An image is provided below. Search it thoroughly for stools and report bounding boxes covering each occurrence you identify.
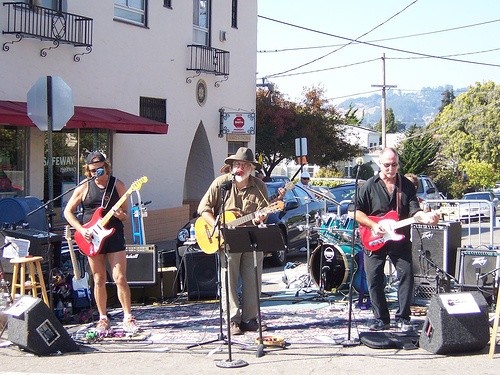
[9,256,50,308]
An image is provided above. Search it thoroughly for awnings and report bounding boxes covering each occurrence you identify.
[0,100,168,134]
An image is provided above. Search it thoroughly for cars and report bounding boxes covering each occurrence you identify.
[457,192,499,221]
[175,180,326,266]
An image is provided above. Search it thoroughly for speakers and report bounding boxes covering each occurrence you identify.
[456,246,500,300]
[103,243,157,284]
[419,290,490,354]
[183,251,220,299]
[7,295,81,354]
[410,222,461,306]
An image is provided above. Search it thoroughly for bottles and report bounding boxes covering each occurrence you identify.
[57,298,64,318]
[189,224,195,238]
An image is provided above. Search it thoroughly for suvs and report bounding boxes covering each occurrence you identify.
[414,175,441,212]
[272,175,290,183]
[324,185,359,215]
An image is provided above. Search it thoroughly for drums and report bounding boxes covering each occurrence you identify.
[309,241,362,291]
[318,211,342,244]
[338,212,361,245]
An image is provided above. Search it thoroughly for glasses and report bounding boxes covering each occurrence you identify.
[90,164,105,173]
[302,178,310,180]
[383,162,398,168]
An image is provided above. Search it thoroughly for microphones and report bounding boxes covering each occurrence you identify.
[357,159,362,171]
[221,181,232,190]
[96,168,104,176]
[475,268,480,284]
[231,169,244,176]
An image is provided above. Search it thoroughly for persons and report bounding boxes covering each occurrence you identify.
[347,147,439,331]
[197,147,286,335]
[64,152,143,333]
[404,173,418,186]
[0,175,21,191]
[296,170,322,198]
[256,171,263,180]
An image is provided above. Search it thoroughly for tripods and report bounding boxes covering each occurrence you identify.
[286,200,313,288]
[186,178,248,349]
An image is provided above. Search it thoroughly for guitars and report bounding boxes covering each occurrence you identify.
[64,223,93,309]
[194,200,287,256]
[353,249,387,297]
[357,206,457,253]
[74,175,149,258]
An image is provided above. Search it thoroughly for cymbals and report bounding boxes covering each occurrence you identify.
[306,188,343,207]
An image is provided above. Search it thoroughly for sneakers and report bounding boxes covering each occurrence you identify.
[94,317,112,332]
[122,314,141,333]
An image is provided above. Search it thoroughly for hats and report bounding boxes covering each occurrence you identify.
[224,146,259,166]
[86,151,105,165]
[300,171,310,178]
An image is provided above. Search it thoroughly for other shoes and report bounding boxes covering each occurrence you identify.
[368,319,390,331]
[230,321,243,335]
[396,318,412,327]
[240,319,268,332]
